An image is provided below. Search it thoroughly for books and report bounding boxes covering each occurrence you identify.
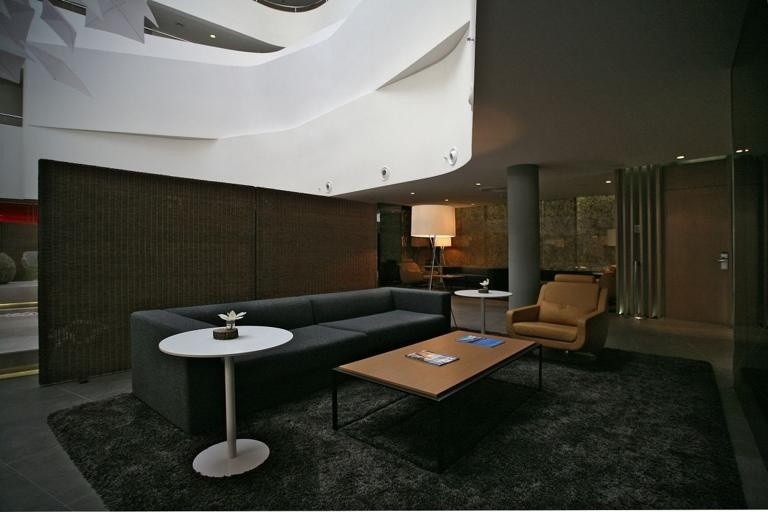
[456,332,507,348]
[404,349,459,367]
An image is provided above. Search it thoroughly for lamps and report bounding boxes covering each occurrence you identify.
[411,202,463,294]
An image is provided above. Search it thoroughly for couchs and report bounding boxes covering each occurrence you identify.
[131,286,455,426]
[506,274,617,364]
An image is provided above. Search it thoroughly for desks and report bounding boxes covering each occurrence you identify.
[330,330,544,425]
[452,287,514,336]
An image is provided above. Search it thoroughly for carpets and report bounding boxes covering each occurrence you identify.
[40,346,742,509]
